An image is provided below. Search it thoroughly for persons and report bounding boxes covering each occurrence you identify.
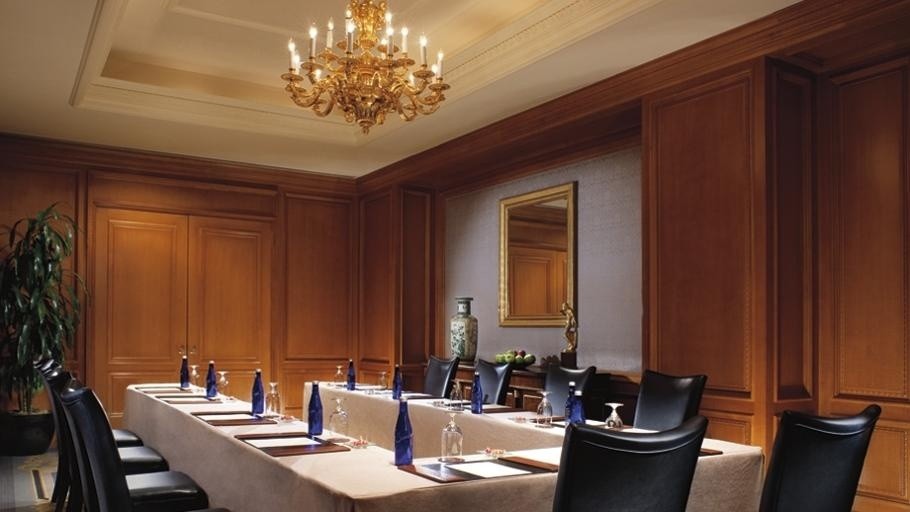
[560,303,580,350]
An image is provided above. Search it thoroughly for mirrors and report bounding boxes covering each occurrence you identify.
[497,181,579,328]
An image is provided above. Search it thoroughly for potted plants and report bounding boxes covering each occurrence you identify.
[0,197,91,458]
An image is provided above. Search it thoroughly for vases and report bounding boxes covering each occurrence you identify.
[449,296,479,365]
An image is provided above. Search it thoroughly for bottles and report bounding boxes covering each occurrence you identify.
[393,364,402,400]
[570,390,585,424]
[395,395,413,465]
[180,356,189,387]
[471,372,482,414]
[347,359,355,390]
[252,369,264,413]
[308,380,323,435]
[565,382,576,425]
[206,361,217,397]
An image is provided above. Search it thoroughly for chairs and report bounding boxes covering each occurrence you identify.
[477,358,516,404]
[757,402,880,509]
[552,413,711,512]
[541,361,599,413]
[423,356,461,395]
[632,368,708,430]
[29,351,210,512]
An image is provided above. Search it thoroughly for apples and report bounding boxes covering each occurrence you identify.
[495,349,536,365]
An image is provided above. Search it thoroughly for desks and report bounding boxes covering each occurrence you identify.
[424,392,763,457]
[422,448,765,512]
[124,380,766,511]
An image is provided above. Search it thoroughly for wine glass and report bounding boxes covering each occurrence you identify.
[604,402,624,432]
[334,365,344,389]
[536,391,553,427]
[377,371,388,395]
[189,365,201,392]
[440,413,463,462]
[264,382,282,415]
[217,371,231,402]
[449,380,464,411]
[327,397,350,440]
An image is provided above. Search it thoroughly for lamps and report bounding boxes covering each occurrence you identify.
[280,1,450,138]
